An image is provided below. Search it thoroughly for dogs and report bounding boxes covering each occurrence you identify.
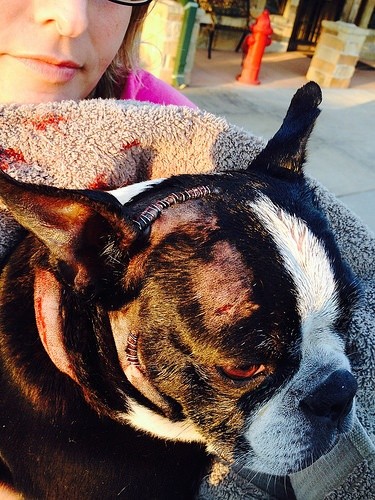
[0,81,362,500]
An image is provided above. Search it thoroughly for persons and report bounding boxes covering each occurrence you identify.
[1,0,206,121]
